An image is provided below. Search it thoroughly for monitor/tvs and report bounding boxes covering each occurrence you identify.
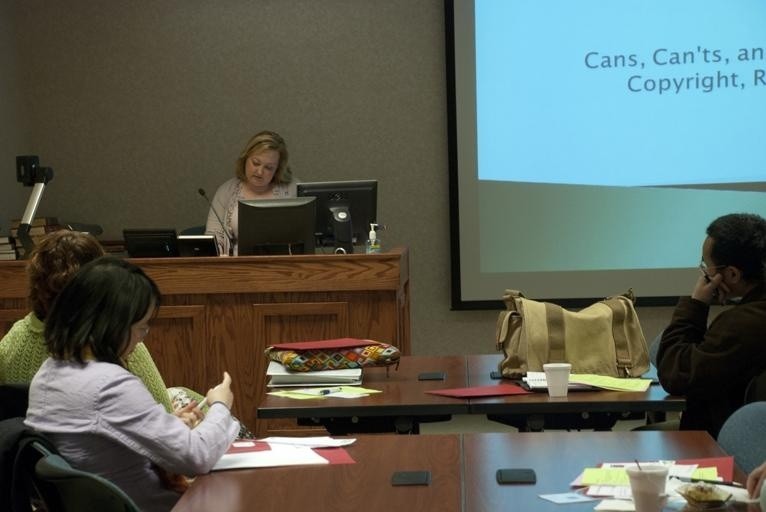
[122,228,182,259]
[238,196,317,256]
[297,180,377,254]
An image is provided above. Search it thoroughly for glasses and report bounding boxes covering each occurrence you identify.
[699,256,725,279]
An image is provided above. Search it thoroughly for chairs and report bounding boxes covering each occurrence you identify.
[649,321,711,368]
[0,383,29,420]
[717,400,766,475]
[24,441,138,512]
[179,224,206,235]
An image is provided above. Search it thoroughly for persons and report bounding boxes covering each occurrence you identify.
[631,213,766,441]
[748,461,765,512]
[21,254,243,512]
[0,227,254,475]
[206,131,305,257]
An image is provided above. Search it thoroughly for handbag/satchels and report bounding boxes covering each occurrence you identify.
[496,289,649,379]
[264,339,400,372]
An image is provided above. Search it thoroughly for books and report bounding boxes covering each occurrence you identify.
[1,217,59,259]
[266,360,363,387]
[517,370,592,391]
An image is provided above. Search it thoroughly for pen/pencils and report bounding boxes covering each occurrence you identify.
[669,476,743,487]
[701,270,719,298]
[198,398,207,410]
[320,388,342,395]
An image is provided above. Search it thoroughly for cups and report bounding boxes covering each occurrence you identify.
[541,360,572,398]
[624,463,671,511]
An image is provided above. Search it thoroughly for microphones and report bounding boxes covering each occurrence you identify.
[198,188,233,256]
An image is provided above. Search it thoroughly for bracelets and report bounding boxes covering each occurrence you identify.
[212,400,231,410]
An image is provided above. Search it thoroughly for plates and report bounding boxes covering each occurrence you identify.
[652,492,738,510]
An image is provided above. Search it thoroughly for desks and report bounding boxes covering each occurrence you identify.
[468,353,685,433]
[256,353,468,434]
[463,430,749,512]
[171,434,462,511]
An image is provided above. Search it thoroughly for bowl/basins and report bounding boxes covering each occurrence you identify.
[675,482,732,506]
